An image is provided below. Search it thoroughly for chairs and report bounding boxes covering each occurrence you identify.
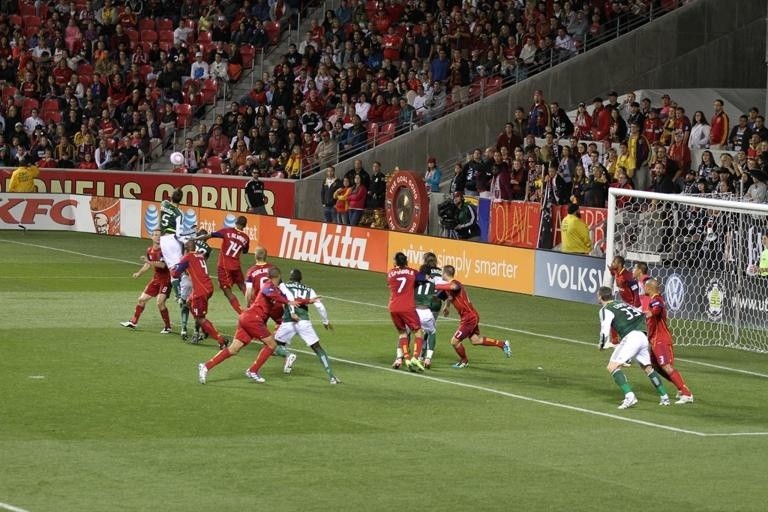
[1,2,287,127]
[471,76,502,98]
[361,0,407,41]
[367,122,397,146]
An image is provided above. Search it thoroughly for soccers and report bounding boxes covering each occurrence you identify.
[169,151,185,166]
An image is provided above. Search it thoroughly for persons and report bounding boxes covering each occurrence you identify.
[639,280,694,405]
[274,269,341,383]
[594,283,670,409]
[199,266,321,382]
[1,1,767,371]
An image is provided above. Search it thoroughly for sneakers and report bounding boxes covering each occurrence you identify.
[392,340,512,373]
[618,385,694,410]
[121,321,342,385]
